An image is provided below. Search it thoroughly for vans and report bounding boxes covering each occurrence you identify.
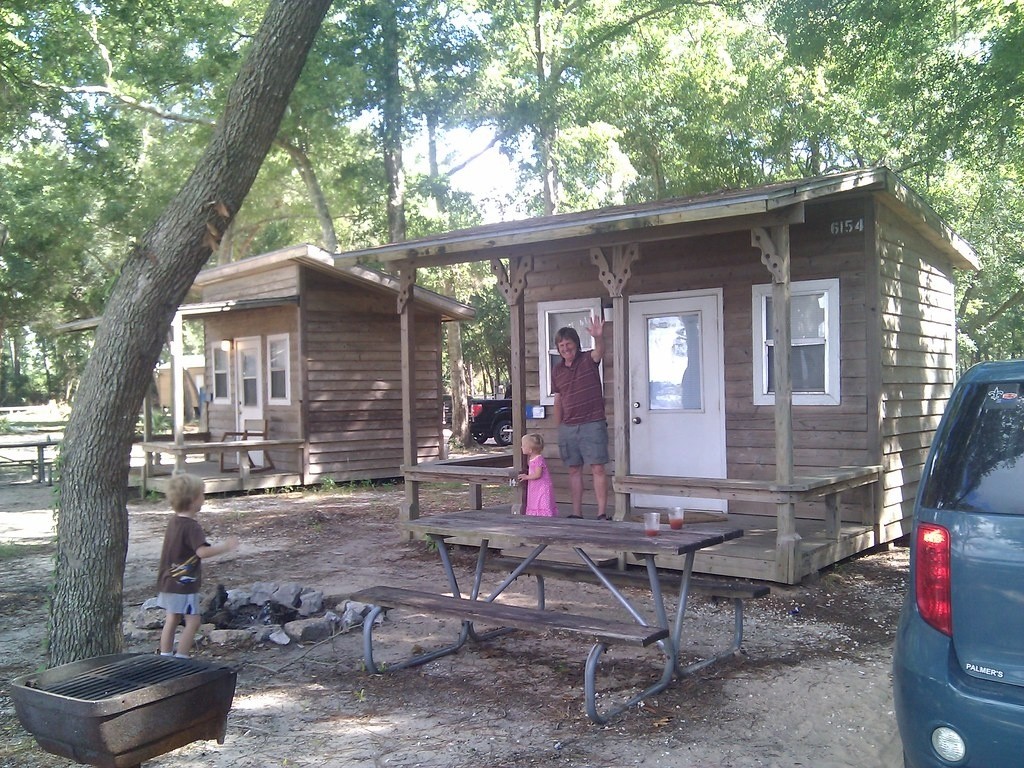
[892,358,1024,768]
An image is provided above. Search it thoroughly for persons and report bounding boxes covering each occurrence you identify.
[158,471,243,660]
[517,433,559,518]
[550,314,610,521]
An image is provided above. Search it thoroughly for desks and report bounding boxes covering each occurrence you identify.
[0,440,62,484]
[392,507,744,686]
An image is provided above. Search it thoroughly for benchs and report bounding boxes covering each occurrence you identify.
[350,585,669,724]
[477,557,771,677]
[0,459,55,487]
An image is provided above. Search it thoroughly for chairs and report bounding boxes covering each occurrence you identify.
[220,418,276,473]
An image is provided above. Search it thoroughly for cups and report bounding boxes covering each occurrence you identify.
[668,507,684,530]
[643,512,661,537]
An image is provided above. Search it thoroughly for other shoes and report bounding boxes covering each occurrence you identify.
[597,514,608,520]
[567,514,583,519]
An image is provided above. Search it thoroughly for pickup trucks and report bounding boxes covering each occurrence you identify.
[442,382,512,448]
